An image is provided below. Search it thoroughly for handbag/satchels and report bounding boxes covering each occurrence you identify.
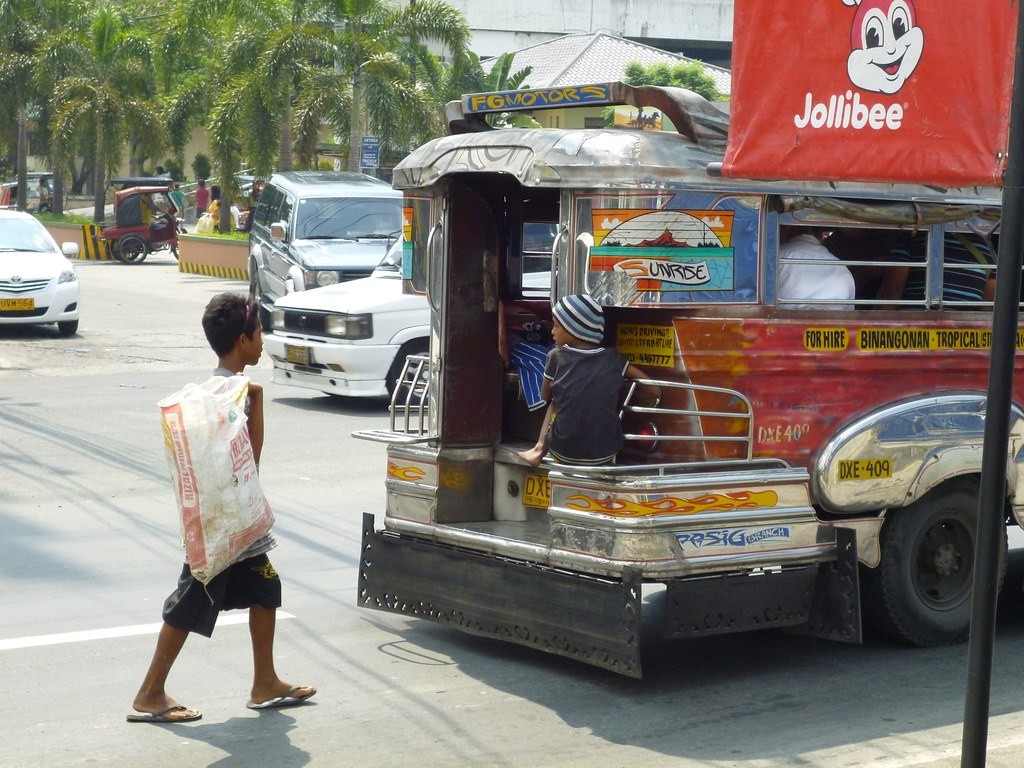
[195,213,214,233]
[159,375,274,585]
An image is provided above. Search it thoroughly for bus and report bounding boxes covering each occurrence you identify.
[358,81,1024,679]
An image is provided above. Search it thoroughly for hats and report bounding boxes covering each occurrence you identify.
[551,294,605,345]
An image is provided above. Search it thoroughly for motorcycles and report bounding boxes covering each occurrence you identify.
[100,185,188,264]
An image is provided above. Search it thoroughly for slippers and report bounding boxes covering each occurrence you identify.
[508,449,534,469]
[127,706,203,721]
[247,686,317,708]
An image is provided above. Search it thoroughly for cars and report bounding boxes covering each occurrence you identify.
[0,208,82,336]
[264,230,431,410]
[19,171,264,232]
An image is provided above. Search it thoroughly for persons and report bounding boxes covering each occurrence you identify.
[127,291,318,723]
[498,269,555,410]
[142,196,163,231]
[167,184,185,220]
[38,179,49,201]
[155,166,166,179]
[193,179,209,219]
[214,200,241,232]
[778,225,856,310]
[874,230,998,312]
[208,185,221,230]
[825,225,895,309]
[518,292,665,469]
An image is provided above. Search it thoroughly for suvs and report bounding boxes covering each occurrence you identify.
[246,171,407,333]
